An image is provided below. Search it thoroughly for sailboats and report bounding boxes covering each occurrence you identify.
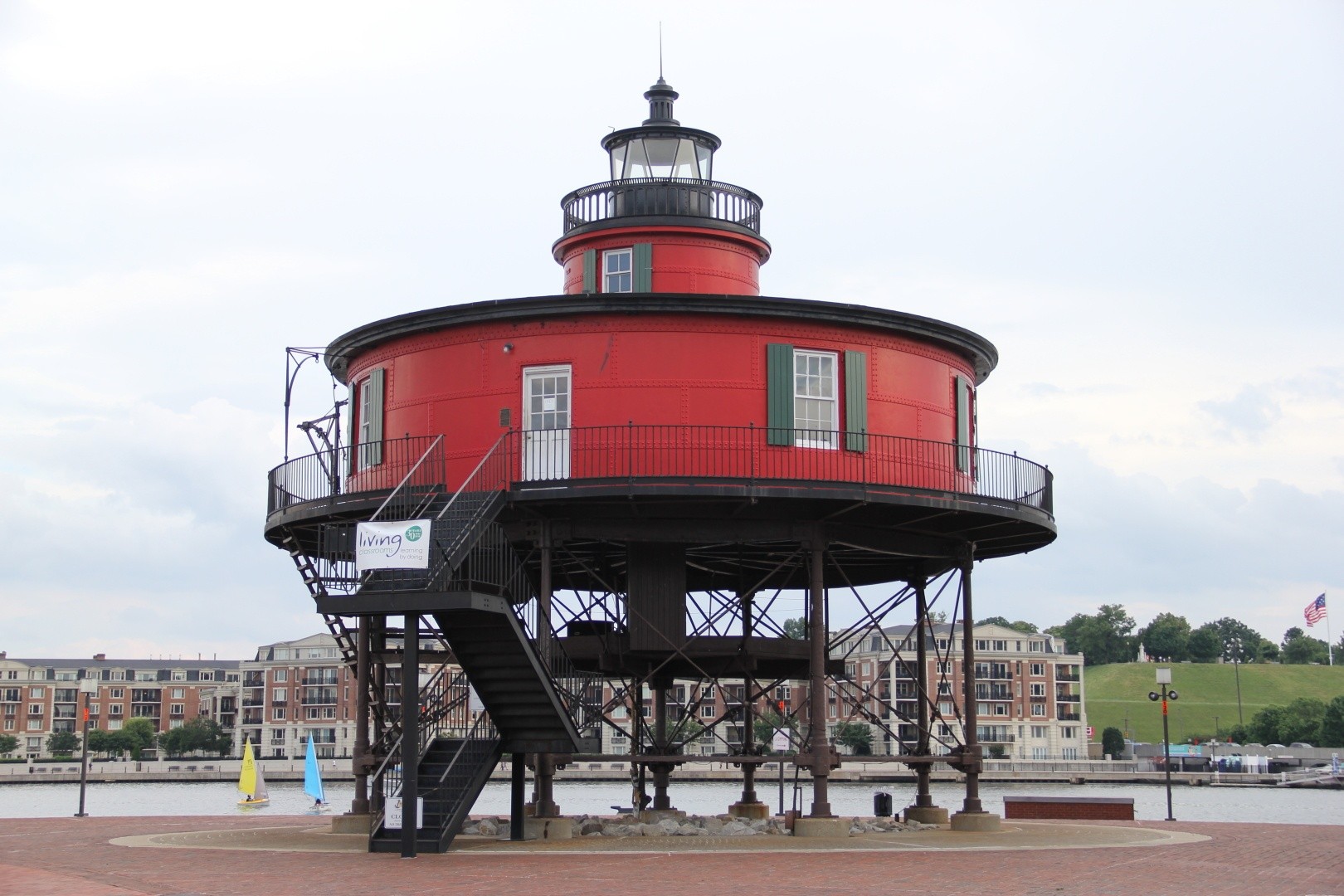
[236,735,271,808]
[303,730,332,813]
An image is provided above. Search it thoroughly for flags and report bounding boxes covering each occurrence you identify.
[1304,593,1327,627]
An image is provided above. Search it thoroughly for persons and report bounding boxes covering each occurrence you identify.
[247,795,253,802]
[89,762,92,772]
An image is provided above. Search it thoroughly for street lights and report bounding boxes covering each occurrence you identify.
[1148,668,1179,822]
[74,677,99,817]
